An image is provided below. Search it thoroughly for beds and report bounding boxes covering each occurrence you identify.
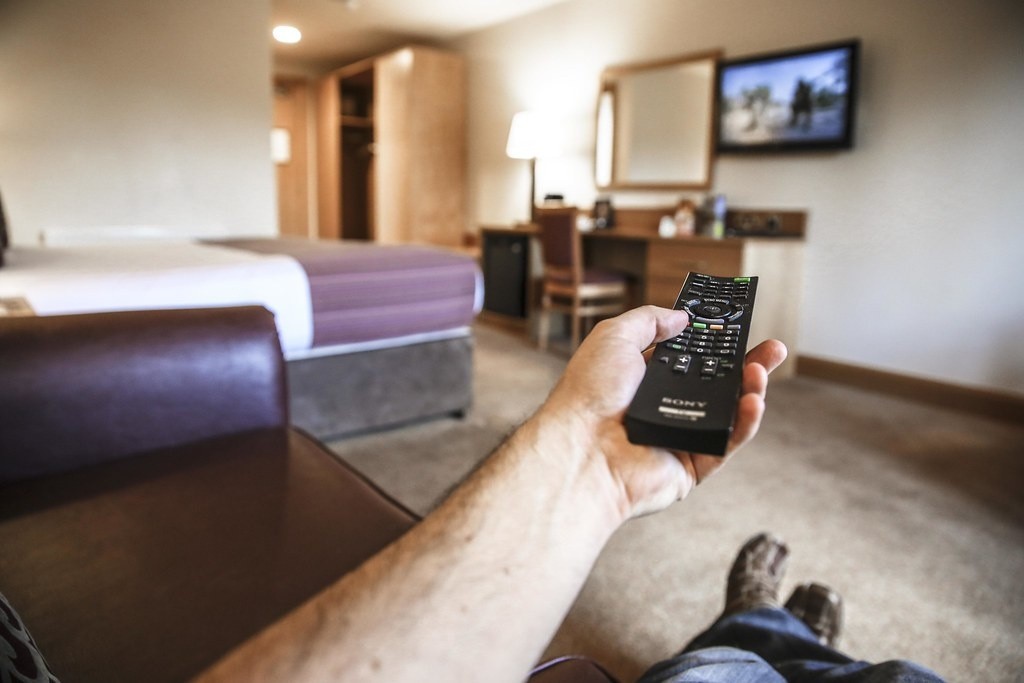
[0,191,486,439]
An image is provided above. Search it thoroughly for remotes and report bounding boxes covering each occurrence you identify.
[621,269,758,458]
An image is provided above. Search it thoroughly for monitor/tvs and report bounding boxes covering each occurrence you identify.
[706,35,863,154]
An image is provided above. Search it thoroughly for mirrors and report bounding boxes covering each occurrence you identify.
[593,48,725,195]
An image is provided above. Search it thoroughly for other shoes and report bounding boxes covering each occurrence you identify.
[784,584,840,650]
[725,534,788,612]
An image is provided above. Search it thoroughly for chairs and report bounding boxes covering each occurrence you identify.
[527,200,628,359]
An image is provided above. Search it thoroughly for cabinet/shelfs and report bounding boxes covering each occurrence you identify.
[315,37,470,251]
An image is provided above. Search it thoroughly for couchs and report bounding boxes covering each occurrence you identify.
[0,302,617,683]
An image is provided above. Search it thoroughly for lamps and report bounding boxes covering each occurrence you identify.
[498,107,555,230]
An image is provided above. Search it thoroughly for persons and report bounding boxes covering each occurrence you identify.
[191,305,945,683]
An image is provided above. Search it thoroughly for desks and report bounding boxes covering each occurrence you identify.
[513,201,742,384]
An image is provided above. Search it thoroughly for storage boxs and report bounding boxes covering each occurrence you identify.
[474,226,527,324]
